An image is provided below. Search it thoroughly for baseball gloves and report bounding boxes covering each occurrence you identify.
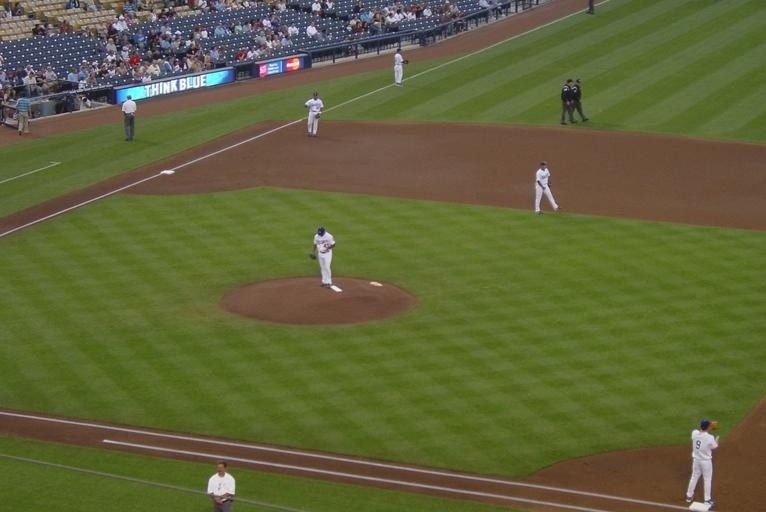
[315,113,320,118]
[308,253,317,260]
[404,60,408,64]
[711,421,719,429]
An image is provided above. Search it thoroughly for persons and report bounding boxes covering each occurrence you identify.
[0,0,206,137]
[304,92,324,138]
[534,160,561,215]
[560,79,576,125]
[310,227,335,288]
[393,47,408,87]
[204,1,498,65]
[586,0,597,16]
[121,96,136,142]
[570,79,590,123]
[686,420,721,503]
[205,460,237,512]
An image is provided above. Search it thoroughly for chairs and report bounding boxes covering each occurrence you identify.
[0,1,488,86]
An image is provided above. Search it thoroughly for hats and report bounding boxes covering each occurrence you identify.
[700,419,712,431]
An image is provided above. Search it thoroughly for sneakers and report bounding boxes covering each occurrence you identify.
[704,500,715,510]
[686,496,693,503]
